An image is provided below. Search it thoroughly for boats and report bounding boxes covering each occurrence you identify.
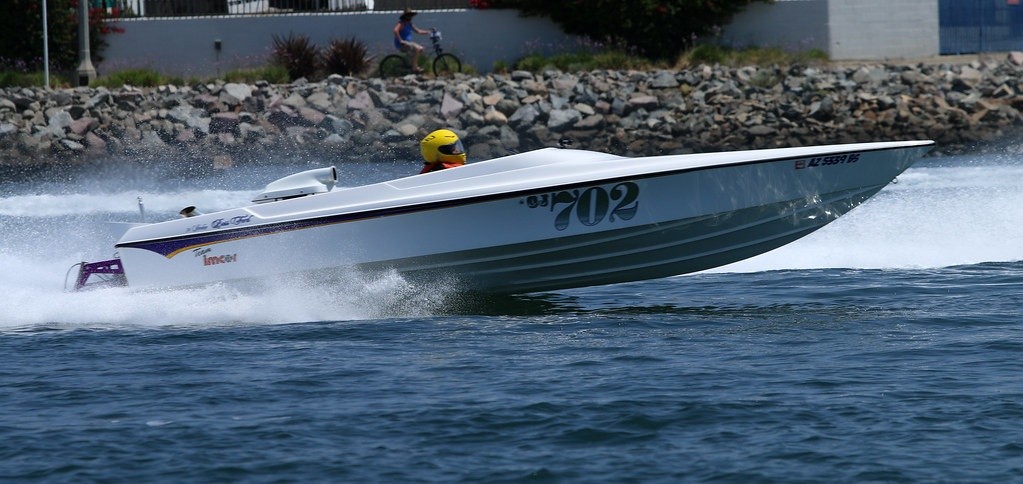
[76,141,936,298]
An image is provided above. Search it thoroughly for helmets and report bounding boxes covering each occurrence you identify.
[419,129,466,164]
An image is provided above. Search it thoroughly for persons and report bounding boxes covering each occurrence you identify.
[393,7,432,74]
[419,129,467,175]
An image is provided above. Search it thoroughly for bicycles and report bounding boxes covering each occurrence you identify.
[375,29,466,81]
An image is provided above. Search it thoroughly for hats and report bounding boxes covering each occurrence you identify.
[400,7,417,20]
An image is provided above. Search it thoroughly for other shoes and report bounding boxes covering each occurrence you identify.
[412,67,424,74]
